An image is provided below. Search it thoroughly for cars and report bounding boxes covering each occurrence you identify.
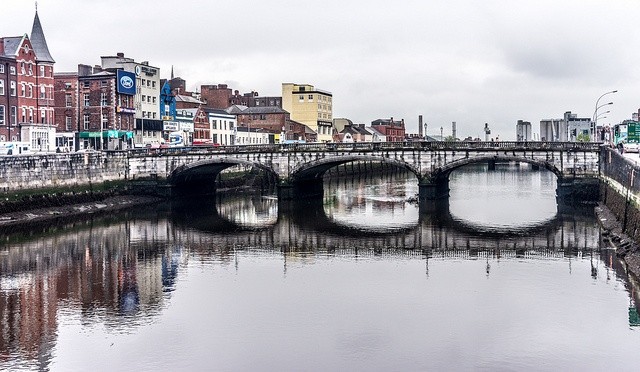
[624,144,639,153]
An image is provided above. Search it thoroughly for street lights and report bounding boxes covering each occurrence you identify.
[595,116,607,142]
[595,111,610,142]
[595,90,617,142]
[593,102,613,120]
